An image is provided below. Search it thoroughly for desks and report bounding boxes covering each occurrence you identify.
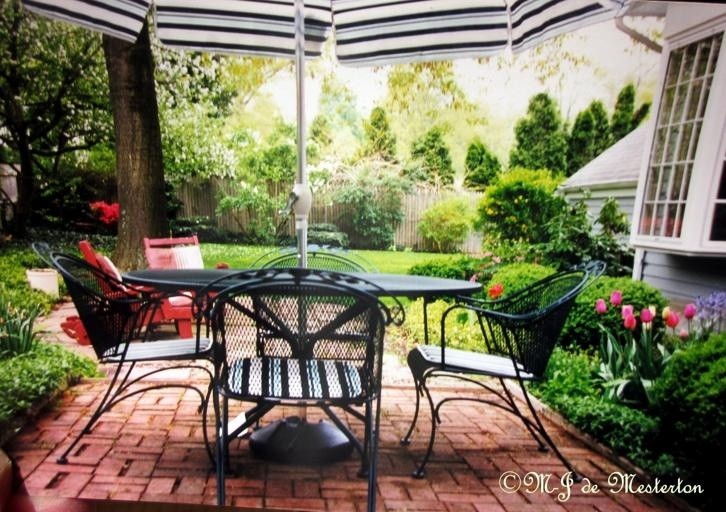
[120,267,481,464]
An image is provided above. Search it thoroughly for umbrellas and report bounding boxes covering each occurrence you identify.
[22,0,634,422]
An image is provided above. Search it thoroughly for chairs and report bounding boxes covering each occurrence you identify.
[79,234,226,338]
[192,268,392,512]
[400,260,606,481]
[49,249,224,470]
[250,244,404,358]
